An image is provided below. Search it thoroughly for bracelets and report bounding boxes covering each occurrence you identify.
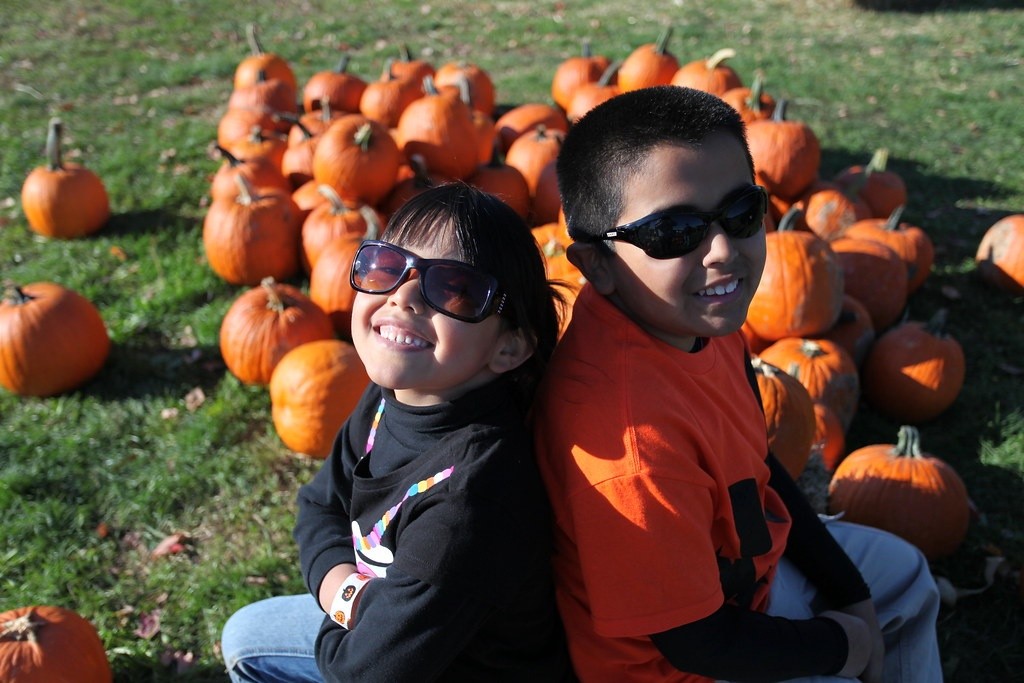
[329,572,375,632]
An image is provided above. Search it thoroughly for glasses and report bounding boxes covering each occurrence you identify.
[350,240,523,326]
[586,185,768,260]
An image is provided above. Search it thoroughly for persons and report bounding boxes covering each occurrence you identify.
[224,182,575,683]
[538,87,941,683]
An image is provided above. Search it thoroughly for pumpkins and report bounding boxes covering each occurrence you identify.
[21,118,112,239]
[0,281,110,396]
[0,604,114,683]
[204,22,1024,571]
